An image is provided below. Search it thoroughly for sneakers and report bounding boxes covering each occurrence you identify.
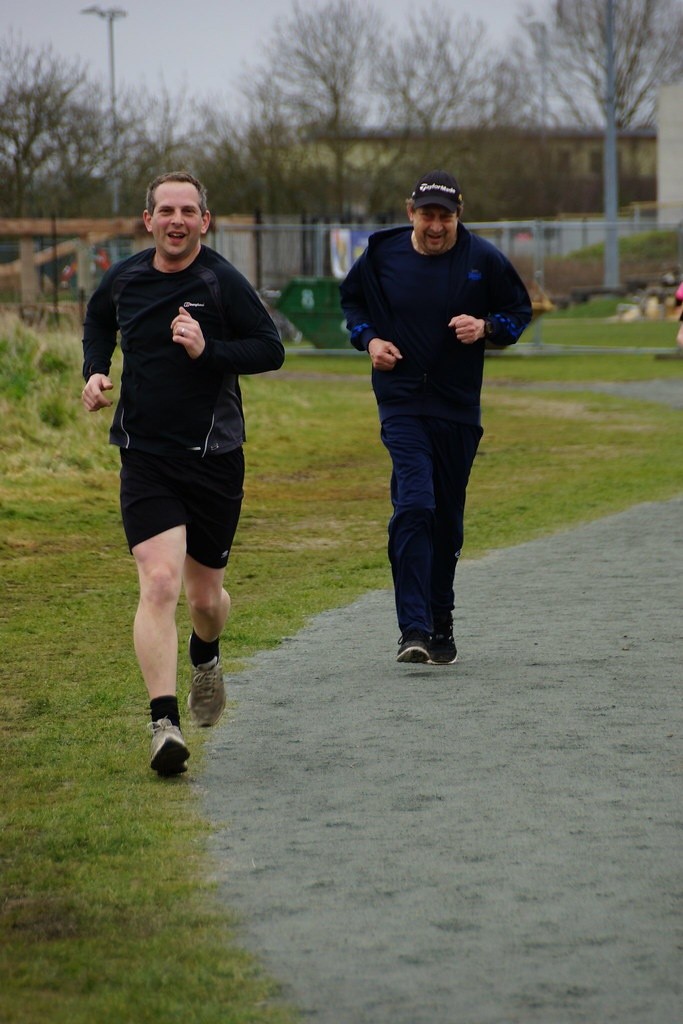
[396,624,458,664]
[147,715,191,779]
[187,634,227,726]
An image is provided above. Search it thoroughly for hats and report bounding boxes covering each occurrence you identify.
[411,169,464,213]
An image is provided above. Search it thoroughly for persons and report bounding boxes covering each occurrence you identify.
[82,172,285,779]
[674,280,683,356]
[341,172,533,664]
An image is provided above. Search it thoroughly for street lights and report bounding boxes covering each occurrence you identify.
[81,6,126,213]
